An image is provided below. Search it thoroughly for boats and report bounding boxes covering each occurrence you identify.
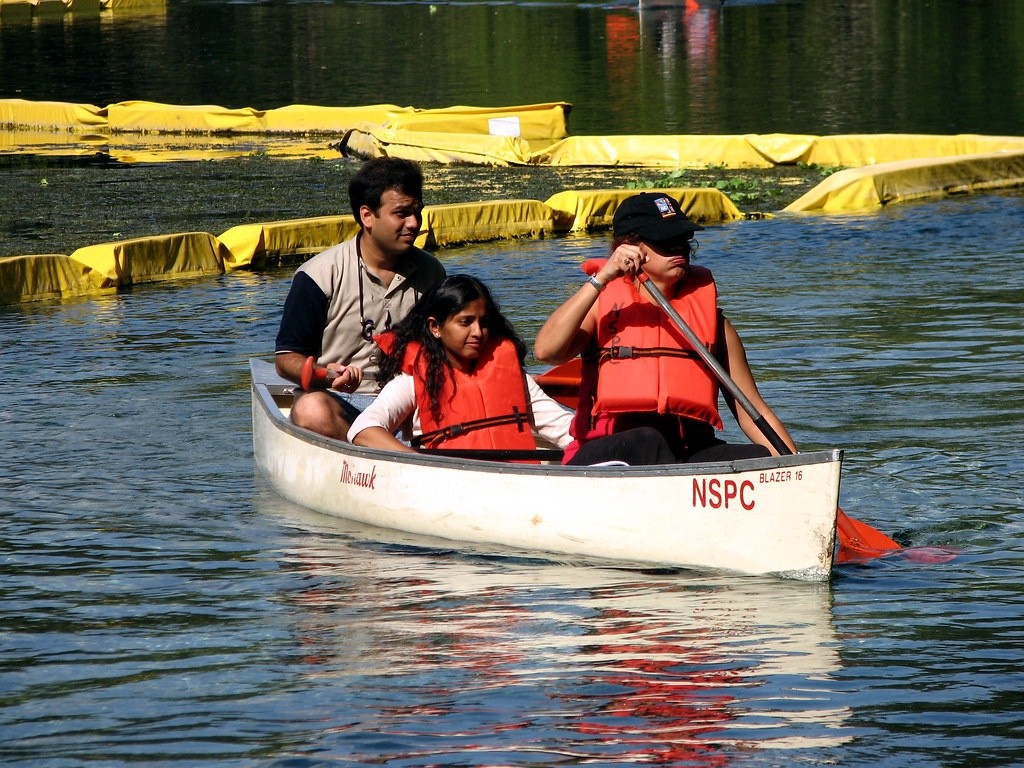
[246,357,845,583]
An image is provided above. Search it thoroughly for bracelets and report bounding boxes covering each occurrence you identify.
[796,451,801,454]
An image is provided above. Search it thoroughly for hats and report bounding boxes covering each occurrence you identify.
[612,192,705,241]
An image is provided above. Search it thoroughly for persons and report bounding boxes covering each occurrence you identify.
[534,192,801,466]
[275,158,576,465]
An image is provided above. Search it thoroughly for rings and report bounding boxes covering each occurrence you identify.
[344,383,351,388]
[624,258,630,265]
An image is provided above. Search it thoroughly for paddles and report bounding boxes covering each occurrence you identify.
[300,355,582,409]
[635,266,902,563]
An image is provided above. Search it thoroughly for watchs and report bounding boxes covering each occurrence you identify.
[586,273,604,292]
